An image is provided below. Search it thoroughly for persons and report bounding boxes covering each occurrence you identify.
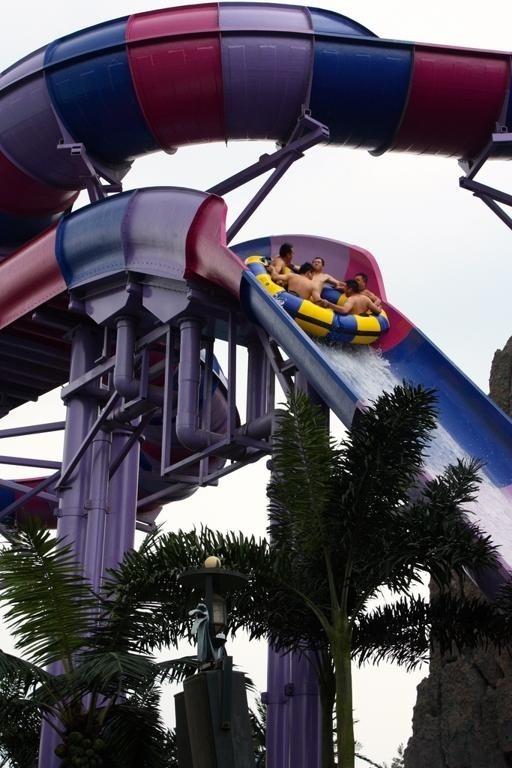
[290,256,340,299]
[266,261,329,308]
[270,242,297,287]
[322,279,383,315]
[334,271,382,309]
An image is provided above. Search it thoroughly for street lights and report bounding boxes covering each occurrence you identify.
[173,556,253,768]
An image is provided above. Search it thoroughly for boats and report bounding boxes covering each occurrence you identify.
[245,255,390,345]
